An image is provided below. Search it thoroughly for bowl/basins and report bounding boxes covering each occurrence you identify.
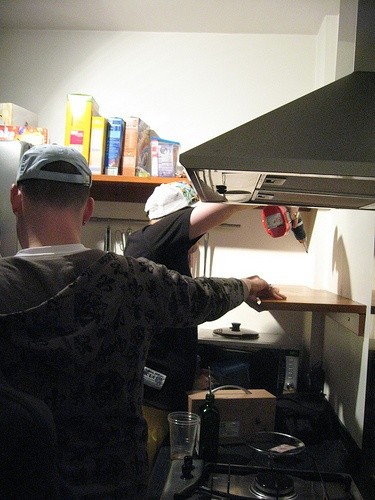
[245,431,305,464]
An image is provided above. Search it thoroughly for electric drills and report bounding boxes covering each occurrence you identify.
[261,205,309,254]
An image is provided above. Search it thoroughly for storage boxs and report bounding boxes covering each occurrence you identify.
[122,116,150,176]
[0,125,48,144]
[104,116,125,176]
[63,92,99,164]
[187,389,276,444]
[157,143,179,177]
[0,102,39,127]
[89,116,108,175]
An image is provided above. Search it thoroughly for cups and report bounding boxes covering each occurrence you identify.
[168,412,199,459]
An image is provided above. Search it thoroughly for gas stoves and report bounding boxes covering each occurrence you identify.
[160,456,363,500]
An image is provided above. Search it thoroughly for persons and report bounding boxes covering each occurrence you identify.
[124,181,300,477]
[0,143,286,500]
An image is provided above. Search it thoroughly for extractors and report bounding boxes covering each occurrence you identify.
[181,72,375,211]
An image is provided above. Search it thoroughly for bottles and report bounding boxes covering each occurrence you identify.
[200,394,220,460]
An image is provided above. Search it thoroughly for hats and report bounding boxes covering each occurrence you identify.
[144,183,192,221]
[16,145,92,190]
